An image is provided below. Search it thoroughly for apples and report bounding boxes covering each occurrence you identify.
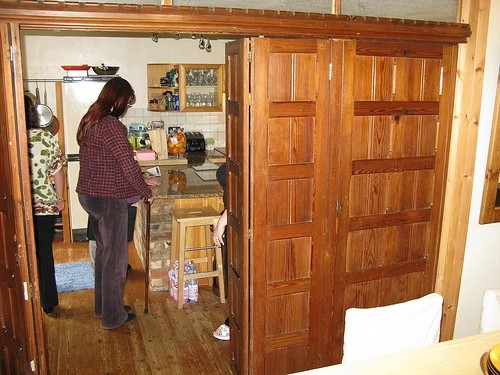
[168,132,186,154]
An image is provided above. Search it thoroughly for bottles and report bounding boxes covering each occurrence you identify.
[127,127,135,148]
[170,260,198,303]
[137,127,146,148]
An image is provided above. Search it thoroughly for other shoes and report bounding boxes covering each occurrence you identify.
[127,313,135,322]
[123,305,132,312]
[213,323,230,340]
[42,306,55,315]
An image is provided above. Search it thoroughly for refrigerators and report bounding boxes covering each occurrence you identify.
[61,81,122,243]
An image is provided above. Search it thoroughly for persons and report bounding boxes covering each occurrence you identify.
[24,94,68,317]
[213,162,232,340]
[77,77,160,330]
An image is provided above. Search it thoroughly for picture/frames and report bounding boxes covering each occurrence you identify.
[479,66,500,224]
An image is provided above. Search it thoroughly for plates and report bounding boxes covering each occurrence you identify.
[61,66,90,70]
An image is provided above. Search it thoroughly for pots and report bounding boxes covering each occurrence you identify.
[25,88,59,137]
[93,63,119,75]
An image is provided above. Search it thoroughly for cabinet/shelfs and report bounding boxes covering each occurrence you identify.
[133,148,227,293]
[22,76,119,243]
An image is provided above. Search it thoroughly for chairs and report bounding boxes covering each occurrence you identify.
[342,293,444,365]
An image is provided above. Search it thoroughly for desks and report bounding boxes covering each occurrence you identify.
[288,329,500,375]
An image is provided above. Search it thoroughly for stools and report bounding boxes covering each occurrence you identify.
[172,206,226,310]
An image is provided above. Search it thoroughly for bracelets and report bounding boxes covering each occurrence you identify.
[56,198,65,201]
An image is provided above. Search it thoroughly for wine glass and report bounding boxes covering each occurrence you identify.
[186,69,217,86]
[186,92,217,107]
